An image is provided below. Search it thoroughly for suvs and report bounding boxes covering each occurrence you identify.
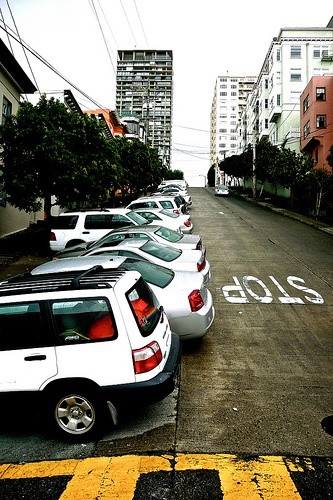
[124,197,188,214]
[50,207,183,250]
[0,265,181,439]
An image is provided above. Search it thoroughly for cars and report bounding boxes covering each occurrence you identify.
[78,238,211,288]
[135,178,193,205]
[214,183,229,195]
[55,224,206,260]
[7,255,216,340]
[132,207,193,234]
